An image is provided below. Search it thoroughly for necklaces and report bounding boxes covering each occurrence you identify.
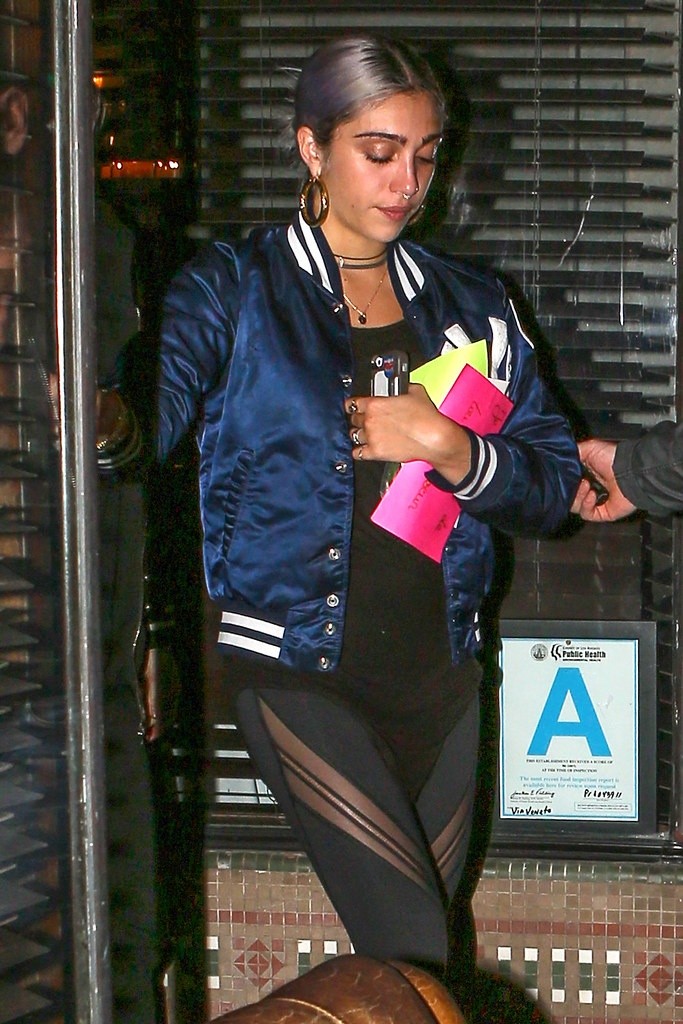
[332,250,390,268]
[342,266,386,325]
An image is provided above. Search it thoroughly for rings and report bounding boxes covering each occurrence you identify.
[358,447,364,462]
[348,397,361,414]
[351,428,363,445]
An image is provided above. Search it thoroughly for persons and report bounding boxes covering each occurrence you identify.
[567,421,683,522]
[98,34,586,981]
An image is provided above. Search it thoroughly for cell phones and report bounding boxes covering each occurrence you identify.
[372,350,409,398]
